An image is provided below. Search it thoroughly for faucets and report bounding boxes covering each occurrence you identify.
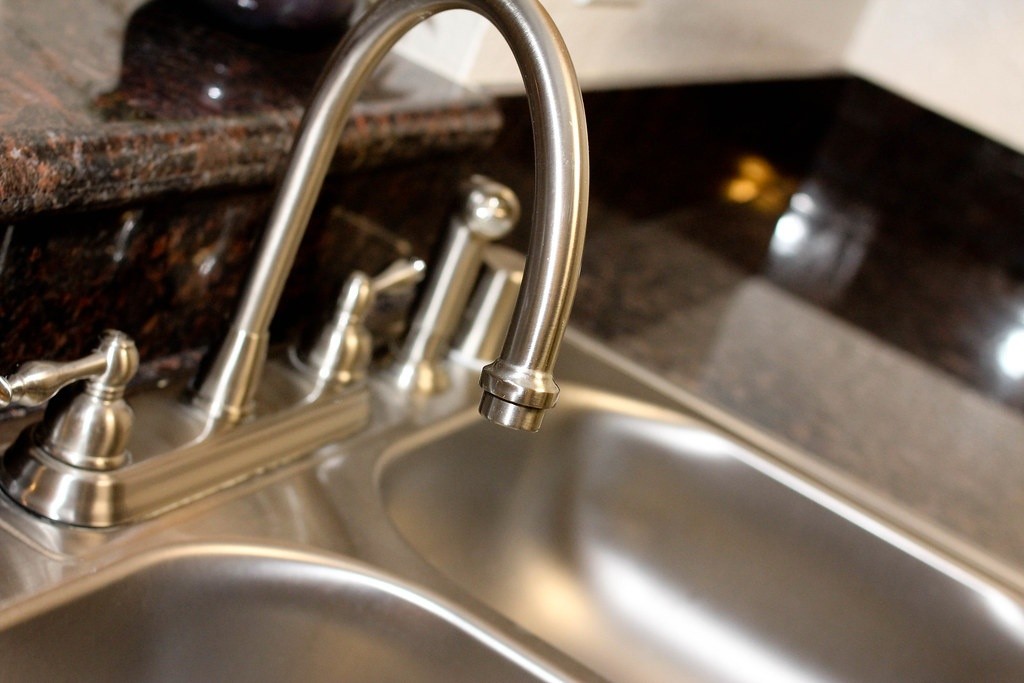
[187,0,592,435]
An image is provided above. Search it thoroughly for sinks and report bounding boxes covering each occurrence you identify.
[370,377,1024,683]
[0,542,572,683]
